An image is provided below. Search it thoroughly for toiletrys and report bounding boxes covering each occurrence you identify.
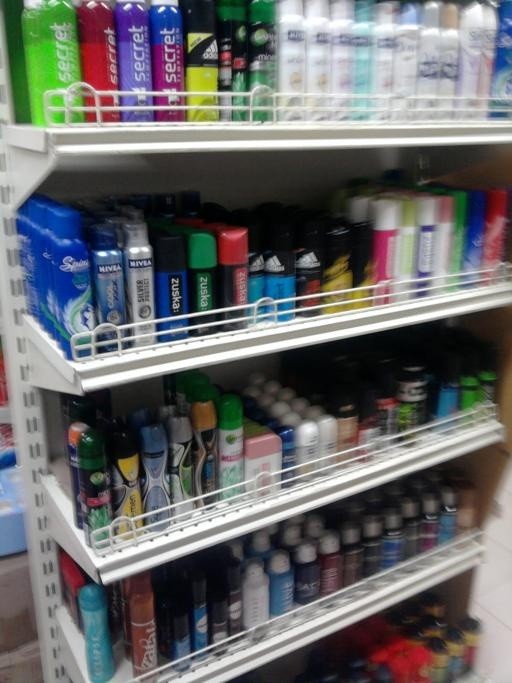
[1,0,512,125]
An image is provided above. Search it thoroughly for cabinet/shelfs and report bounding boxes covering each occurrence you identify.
[1,0,512,683]
